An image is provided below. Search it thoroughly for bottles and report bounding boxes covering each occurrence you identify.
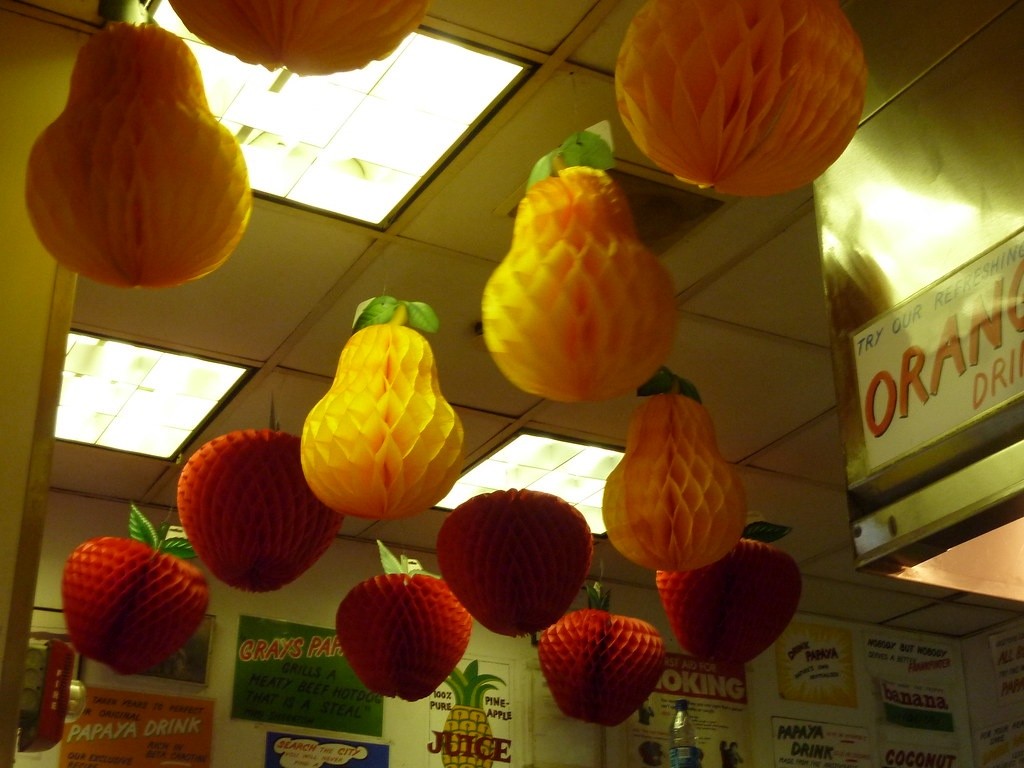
[668,697,701,768]
